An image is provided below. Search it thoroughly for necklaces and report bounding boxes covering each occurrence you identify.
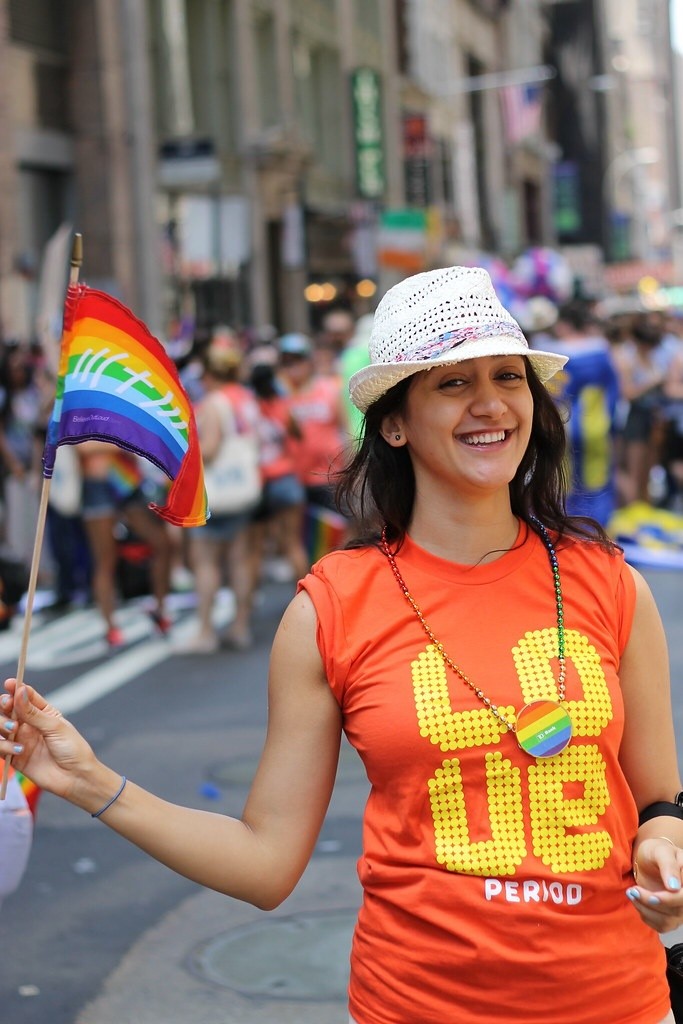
[375,510,574,758]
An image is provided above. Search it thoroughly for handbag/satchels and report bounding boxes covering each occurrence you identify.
[203,388,262,516]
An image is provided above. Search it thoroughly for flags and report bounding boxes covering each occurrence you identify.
[502,83,544,145]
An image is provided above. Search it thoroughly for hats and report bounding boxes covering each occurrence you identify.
[347,265,569,413]
[516,294,559,332]
[277,330,314,355]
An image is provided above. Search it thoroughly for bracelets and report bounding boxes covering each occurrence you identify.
[90,777,128,820]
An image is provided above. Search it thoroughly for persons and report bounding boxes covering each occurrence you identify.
[3,203,681,658]
[0,263,681,1023]
